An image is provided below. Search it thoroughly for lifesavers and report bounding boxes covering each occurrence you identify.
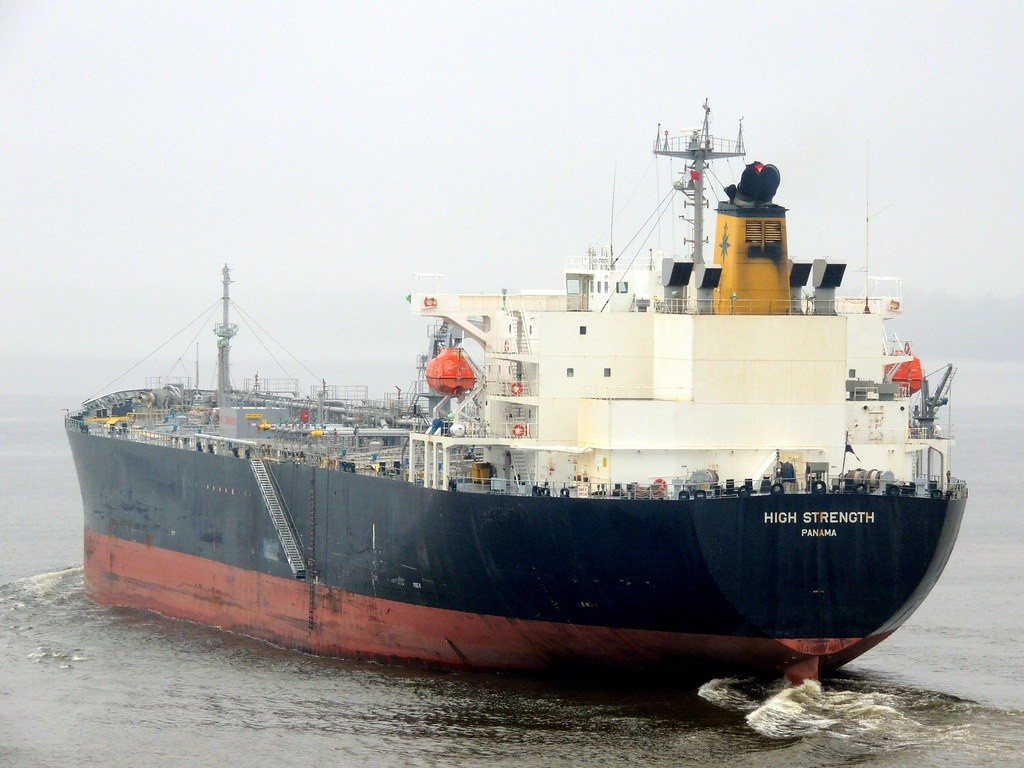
[512,424,525,437]
[904,343,910,354]
[653,479,667,492]
[511,382,523,395]
[424,297,437,308]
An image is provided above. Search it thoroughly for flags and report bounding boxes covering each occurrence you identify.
[685,168,702,182]
[846,440,861,462]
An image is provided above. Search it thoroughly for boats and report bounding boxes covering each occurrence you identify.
[62,97,970,679]
